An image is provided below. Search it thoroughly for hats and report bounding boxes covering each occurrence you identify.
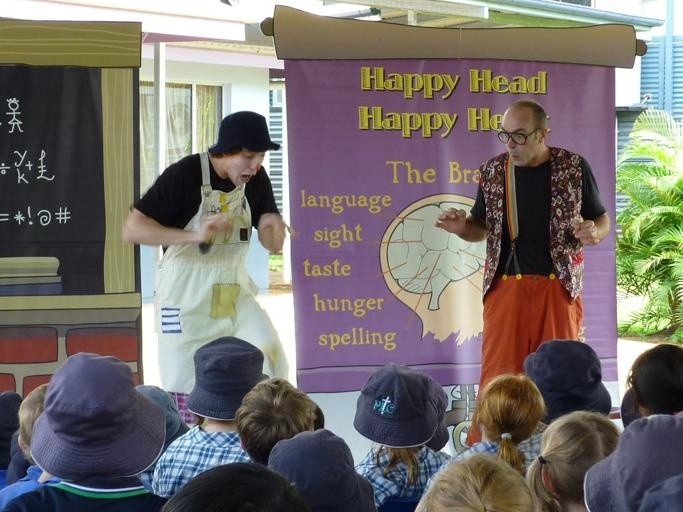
[353,361,450,452]
[620,388,639,425]
[185,336,270,421]
[208,110,280,154]
[29,352,167,480]
[524,340,612,418]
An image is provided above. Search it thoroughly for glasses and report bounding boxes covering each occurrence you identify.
[497,128,540,144]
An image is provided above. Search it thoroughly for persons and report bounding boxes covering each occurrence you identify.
[236,378,318,465]
[435,98,609,447]
[527,412,620,511]
[354,362,453,510]
[453,371,546,478]
[164,461,313,510]
[7,383,48,483]
[629,344,681,427]
[153,335,271,500]
[1,351,169,510]
[0,391,22,471]
[124,111,288,395]
[622,390,641,427]
[416,450,540,511]
[522,340,612,434]
